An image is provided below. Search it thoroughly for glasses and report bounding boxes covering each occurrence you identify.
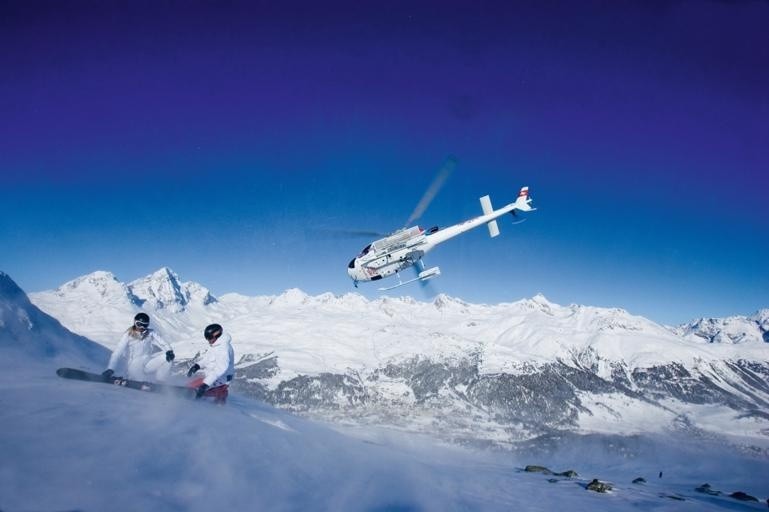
[135,321,149,329]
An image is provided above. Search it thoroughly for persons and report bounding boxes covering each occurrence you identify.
[102,313,175,383]
[188,324,234,404]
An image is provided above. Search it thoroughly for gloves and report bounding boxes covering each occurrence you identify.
[196,383,208,398]
[166,351,174,360]
[102,370,113,377]
[187,364,199,376]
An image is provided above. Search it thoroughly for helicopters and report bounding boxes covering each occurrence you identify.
[300,164,540,293]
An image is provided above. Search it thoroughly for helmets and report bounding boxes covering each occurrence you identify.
[134,313,150,322]
[204,324,222,340]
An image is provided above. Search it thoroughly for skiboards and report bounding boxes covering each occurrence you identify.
[56,368,196,399]
